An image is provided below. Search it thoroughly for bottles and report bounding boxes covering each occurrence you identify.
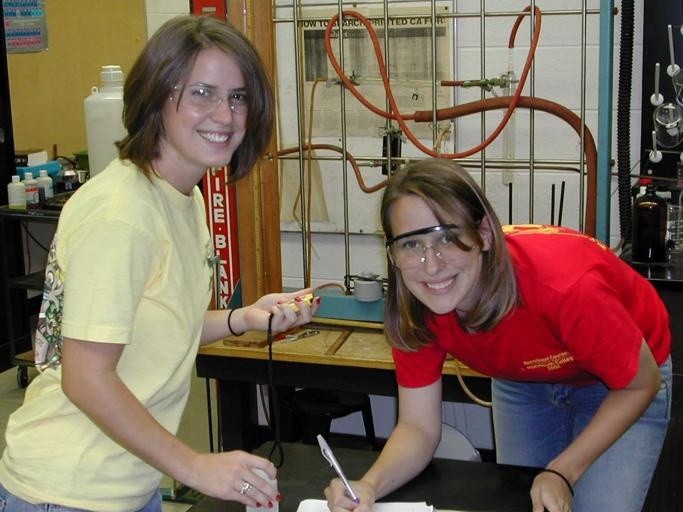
[632,173,682,263]
[243,463,279,512]
[7,170,54,209]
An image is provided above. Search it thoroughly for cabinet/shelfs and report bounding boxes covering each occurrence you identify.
[6,209,65,362]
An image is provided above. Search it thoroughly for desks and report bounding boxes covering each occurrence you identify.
[195,300,495,456]
[182,437,552,512]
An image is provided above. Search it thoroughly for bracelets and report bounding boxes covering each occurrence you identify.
[228,306,245,336]
[543,469,574,499]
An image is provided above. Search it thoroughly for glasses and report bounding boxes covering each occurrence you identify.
[383,218,481,269]
[165,80,249,118]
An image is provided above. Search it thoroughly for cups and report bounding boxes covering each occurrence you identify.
[71,148,88,182]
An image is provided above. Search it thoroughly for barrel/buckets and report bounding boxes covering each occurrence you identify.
[81,62,126,179]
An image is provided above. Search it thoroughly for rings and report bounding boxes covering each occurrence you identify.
[240,482,252,494]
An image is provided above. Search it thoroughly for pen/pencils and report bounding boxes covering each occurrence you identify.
[316,434,359,505]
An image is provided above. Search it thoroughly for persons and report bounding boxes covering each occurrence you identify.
[0,14,320,512]
[324,158,673,512]
